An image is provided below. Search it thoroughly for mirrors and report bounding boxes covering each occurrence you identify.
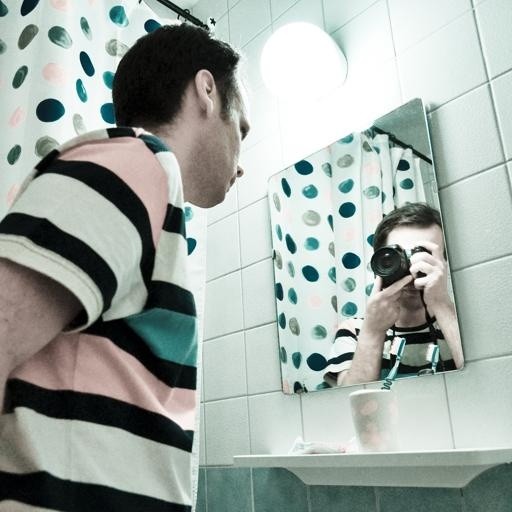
[267,98,466,395]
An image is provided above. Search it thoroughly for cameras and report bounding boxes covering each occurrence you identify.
[371,243,432,288]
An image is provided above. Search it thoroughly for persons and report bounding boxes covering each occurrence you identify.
[0,20,252,511]
[322,200,461,392]
